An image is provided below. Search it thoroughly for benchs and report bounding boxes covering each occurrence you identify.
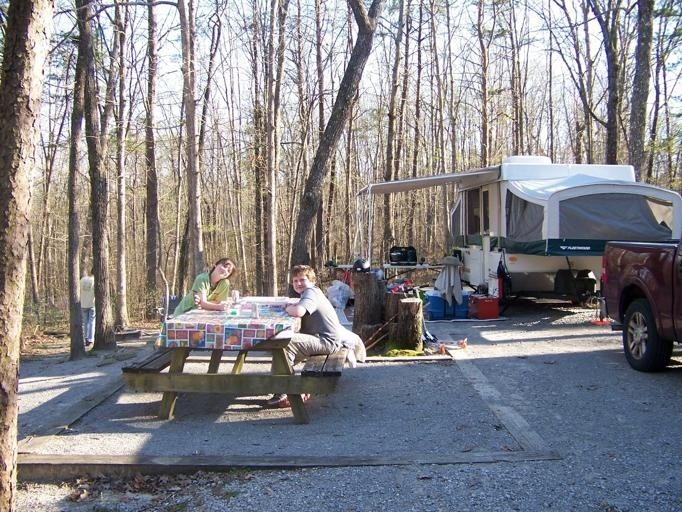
[122,345,349,424]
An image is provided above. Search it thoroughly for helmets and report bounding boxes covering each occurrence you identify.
[352,258,371,272]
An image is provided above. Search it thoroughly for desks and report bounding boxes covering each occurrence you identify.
[382,262,464,291]
[158,296,310,424]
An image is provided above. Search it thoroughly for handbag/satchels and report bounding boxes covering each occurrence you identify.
[389,245,417,266]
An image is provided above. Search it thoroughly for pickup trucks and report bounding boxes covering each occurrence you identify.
[597,239,682,372]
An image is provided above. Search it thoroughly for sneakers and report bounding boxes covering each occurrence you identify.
[266,393,311,408]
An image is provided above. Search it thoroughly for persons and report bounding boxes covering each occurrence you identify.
[173,258,234,318]
[263,265,342,408]
[79,266,97,346]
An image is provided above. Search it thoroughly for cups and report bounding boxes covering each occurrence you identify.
[232,290,239,303]
[251,303,259,319]
